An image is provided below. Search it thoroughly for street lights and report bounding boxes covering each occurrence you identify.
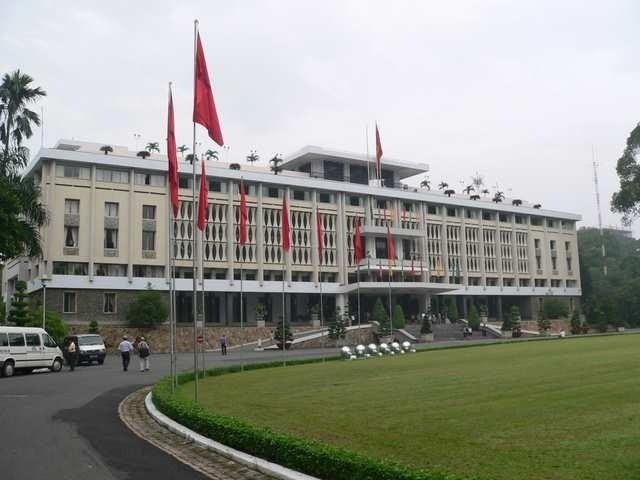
[40,272,55,331]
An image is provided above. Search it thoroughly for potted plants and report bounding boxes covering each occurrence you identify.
[511,304,522,337]
[254,303,268,329]
[309,304,320,320]
[537,311,553,336]
[570,310,581,334]
[374,310,395,345]
[271,320,293,350]
[419,315,434,342]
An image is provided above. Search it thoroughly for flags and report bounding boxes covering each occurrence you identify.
[167,87,181,220]
[192,30,223,146]
[240,176,248,249]
[283,198,290,255]
[317,208,323,264]
[387,226,396,265]
[353,216,364,264]
[376,128,383,165]
[197,160,209,233]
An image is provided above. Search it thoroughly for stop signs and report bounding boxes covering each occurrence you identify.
[197,336,203,343]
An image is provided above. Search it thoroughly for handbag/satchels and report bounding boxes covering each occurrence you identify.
[139,348,149,358]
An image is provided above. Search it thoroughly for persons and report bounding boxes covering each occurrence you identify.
[410,310,448,325]
[221,333,229,356]
[117,335,134,372]
[67,337,78,372]
[137,336,151,372]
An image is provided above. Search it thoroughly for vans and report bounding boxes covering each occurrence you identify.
[0,326,64,378]
[62,333,106,366]
